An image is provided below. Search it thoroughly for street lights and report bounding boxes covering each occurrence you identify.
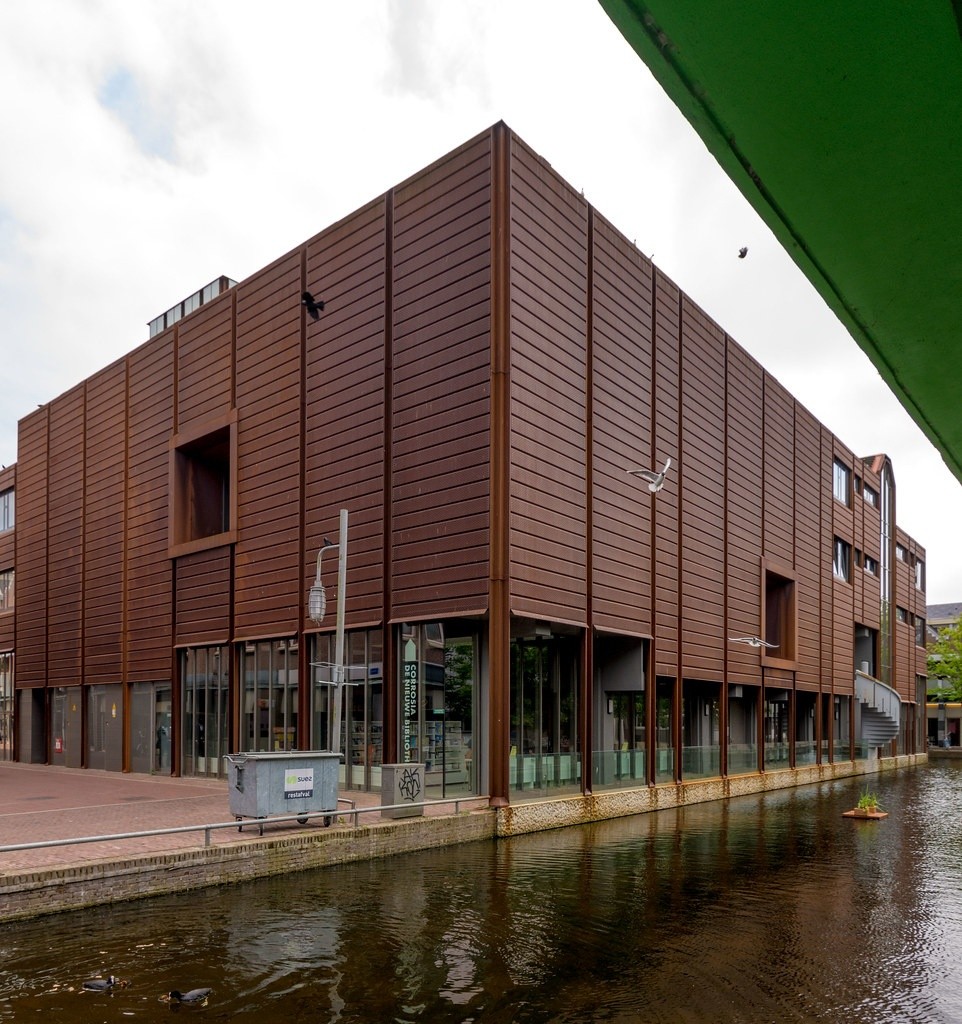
[309,508,348,751]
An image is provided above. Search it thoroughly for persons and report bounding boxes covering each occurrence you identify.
[943,731,952,750]
[465,739,472,771]
[156,726,169,769]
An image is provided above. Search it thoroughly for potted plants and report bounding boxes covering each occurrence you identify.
[854,784,888,815]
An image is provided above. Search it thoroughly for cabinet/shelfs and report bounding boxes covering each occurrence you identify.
[408,721,469,784]
[339,721,383,766]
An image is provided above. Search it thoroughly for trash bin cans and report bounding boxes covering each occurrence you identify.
[222,750,346,835]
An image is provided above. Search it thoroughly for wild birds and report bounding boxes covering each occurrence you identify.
[626,458,672,493]
[738,246,747,258]
[728,636,783,649]
[298,286,327,322]
[324,537,334,547]
